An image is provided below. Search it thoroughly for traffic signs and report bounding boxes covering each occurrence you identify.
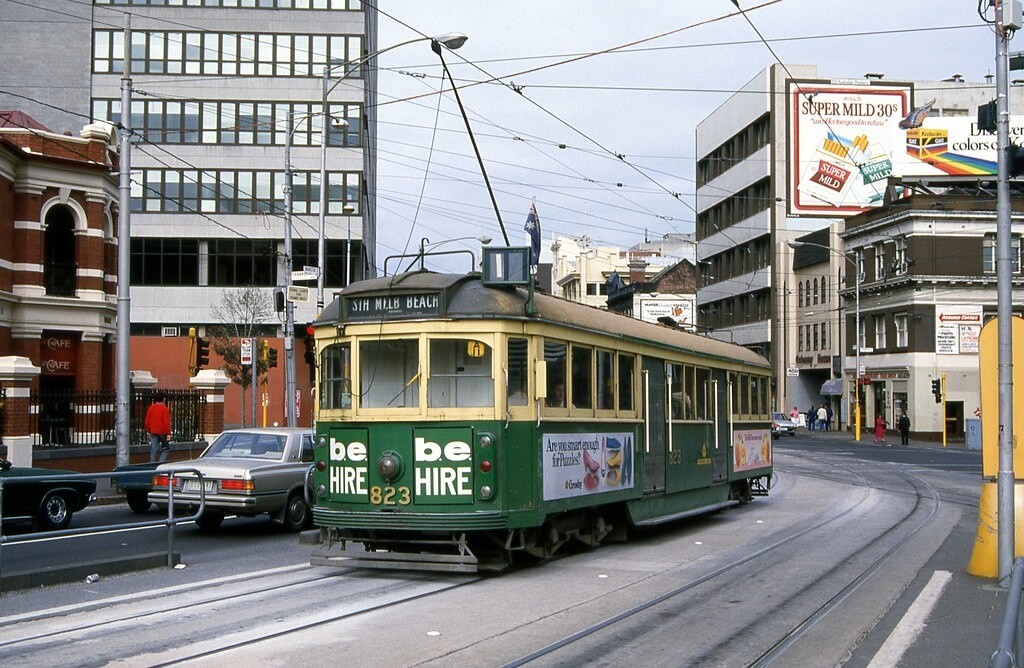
[935,303,983,357]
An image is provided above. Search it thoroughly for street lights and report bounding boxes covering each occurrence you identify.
[786,240,861,442]
[417,236,492,270]
[284,110,349,427]
[317,31,469,316]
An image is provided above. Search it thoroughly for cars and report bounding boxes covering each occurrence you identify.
[0,457,98,532]
[772,411,797,436]
[771,421,781,440]
[148,428,317,533]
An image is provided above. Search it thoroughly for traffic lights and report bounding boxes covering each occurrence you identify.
[304,323,315,364]
[931,379,940,404]
[864,378,871,386]
[858,379,863,385]
[196,337,210,368]
[268,348,278,368]
[853,378,858,399]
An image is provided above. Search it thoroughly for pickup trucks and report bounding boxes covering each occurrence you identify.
[113,457,189,515]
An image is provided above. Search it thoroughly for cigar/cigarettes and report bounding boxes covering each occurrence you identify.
[823,134,869,164]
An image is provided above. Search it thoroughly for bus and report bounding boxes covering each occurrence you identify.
[309,37,778,575]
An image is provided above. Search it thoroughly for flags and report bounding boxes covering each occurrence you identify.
[524,200,541,279]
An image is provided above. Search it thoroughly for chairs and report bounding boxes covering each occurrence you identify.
[303,449,315,462]
[255,443,278,455]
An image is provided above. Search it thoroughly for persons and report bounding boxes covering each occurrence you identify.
[898,414,910,445]
[508,374,528,406]
[817,405,833,432]
[790,407,800,423]
[807,405,818,433]
[874,414,885,443]
[548,381,576,408]
[145,393,171,462]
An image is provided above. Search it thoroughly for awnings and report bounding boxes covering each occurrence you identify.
[820,379,843,395]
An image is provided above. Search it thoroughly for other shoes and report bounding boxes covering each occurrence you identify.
[873,440,878,444]
[881,439,885,442]
